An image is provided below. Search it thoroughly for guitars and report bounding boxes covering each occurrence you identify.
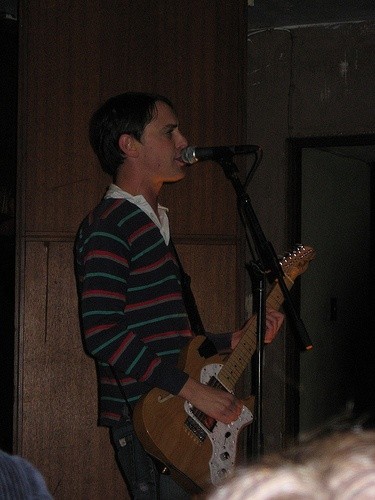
[134,245,315,497]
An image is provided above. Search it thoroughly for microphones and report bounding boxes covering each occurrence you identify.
[180,144,262,164]
